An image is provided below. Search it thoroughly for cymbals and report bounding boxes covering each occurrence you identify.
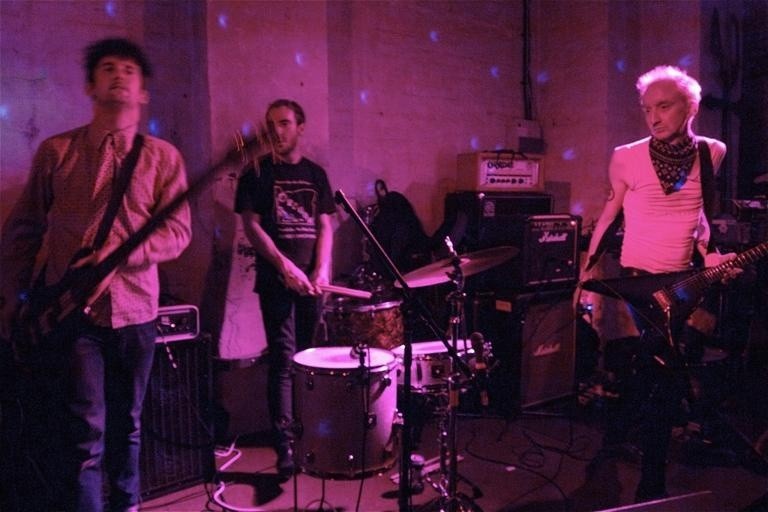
[392,246,521,289]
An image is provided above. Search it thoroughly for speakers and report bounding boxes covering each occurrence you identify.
[445,189,581,408]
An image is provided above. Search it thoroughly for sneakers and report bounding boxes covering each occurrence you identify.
[278,445,295,474]
[616,437,647,467]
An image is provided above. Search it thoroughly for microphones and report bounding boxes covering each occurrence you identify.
[469,331,490,407]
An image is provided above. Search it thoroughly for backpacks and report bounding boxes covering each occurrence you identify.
[375,179,431,272]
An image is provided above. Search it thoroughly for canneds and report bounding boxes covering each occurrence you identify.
[408,453,425,488]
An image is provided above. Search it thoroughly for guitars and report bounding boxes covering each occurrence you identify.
[7,123,285,366]
[577,242,768,367]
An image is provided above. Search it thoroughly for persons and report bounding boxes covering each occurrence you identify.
[0,38,192,512]
[572,66,744,504]
[234,98,337,470]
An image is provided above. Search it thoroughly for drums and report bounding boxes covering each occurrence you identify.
[292,344,400,481]
[393,338,486,394]
[337,291,405,350]
